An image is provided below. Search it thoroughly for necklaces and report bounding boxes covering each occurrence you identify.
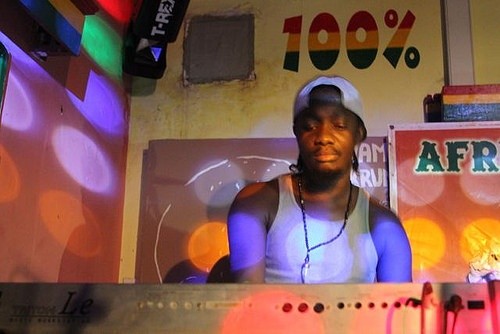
[296,172,353,283]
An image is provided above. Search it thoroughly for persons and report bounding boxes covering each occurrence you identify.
[227,74,413,284]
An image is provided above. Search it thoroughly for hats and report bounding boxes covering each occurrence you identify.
[290,75,370,144]
